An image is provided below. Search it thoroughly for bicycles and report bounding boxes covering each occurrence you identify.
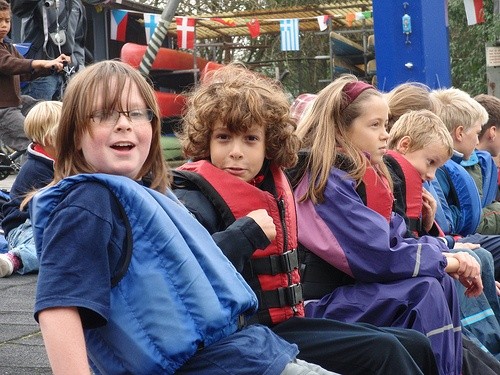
[0,61,77,180]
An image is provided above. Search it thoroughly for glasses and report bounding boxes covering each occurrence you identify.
[87,108,154,129]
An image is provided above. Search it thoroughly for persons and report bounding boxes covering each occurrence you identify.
[0,0,71,153]
[19,60,340,375]
[0,100,63,277]
[11,0,86,101]
[167,59,500,375]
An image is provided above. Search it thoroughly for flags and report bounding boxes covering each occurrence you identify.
[317,15,328,31]
[280,19,299,51]
[110,10,128,42]
[176,18,194,49]
[463,0,486,26]
[143,13,162,44]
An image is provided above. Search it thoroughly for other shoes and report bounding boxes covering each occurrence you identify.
[0,252,23,277]
